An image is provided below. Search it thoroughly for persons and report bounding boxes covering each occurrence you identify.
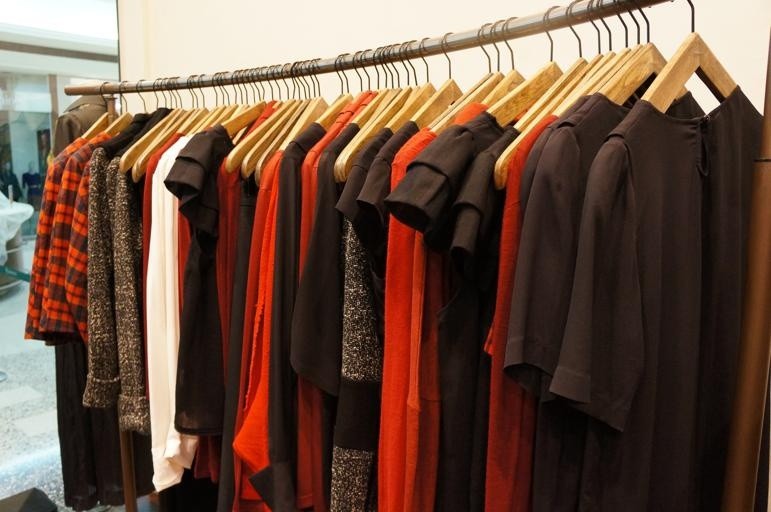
[50,79,111,158]
[39,132,51,173]
[2,162,21,203]
[22,161,44,213]
